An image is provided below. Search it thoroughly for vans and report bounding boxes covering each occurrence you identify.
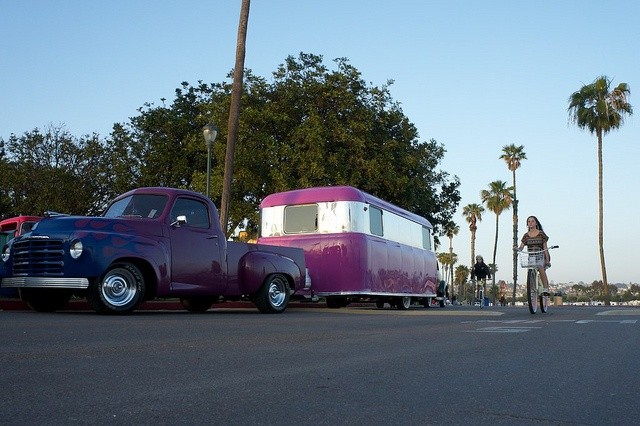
[0,214,40,242]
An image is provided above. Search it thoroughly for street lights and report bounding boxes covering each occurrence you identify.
[202,118,232,202]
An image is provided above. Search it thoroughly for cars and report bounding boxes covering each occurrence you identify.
[376,257,449,307]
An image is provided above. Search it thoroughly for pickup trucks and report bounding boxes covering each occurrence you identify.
[0,184,307,315]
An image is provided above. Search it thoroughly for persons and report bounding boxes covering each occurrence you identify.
[471,255,492,299]
[500,281,507,300]
[513,216,552,293]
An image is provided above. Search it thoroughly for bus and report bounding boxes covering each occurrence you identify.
[259,187,436,310]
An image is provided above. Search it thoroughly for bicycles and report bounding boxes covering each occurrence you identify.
[516,245,559,316]
[471,274,493,308]
[499,291,509,306]
